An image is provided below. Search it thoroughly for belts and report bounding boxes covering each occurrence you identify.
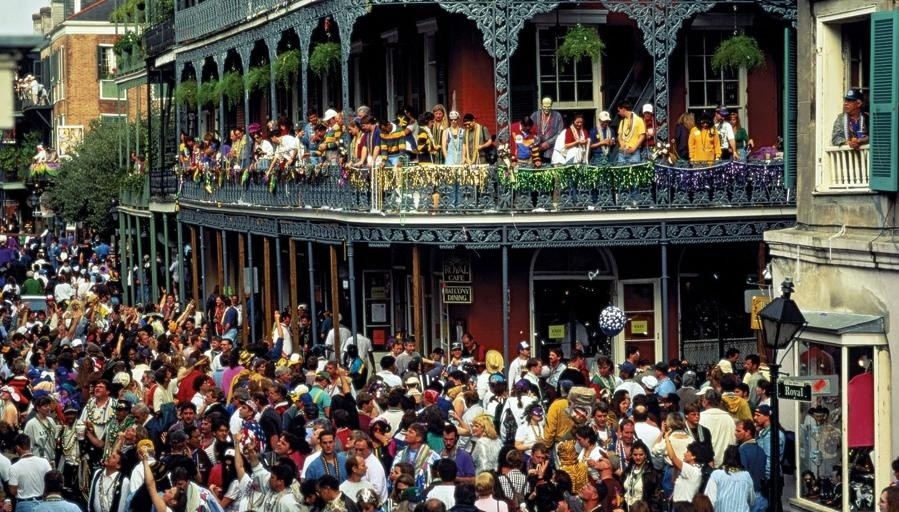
[17,497,43,502]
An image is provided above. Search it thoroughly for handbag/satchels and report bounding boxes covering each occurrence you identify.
[484,143,499,164]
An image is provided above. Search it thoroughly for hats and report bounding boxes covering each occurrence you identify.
[587,474,608,501]
[26,367,80,413]
[616,361,682,401]
[484,341,533,392]
[237,350,255,365]
[239,397,260,415]
[558,378,574,394]
[451,342,463,351]
[136,439,157,452]
[17,320,84,347]
[111,372,131,412]
[404,356,422,385]
[280,304,308,319]
[843,88,864,102]
[755,405,773,415]
[597,110,613,122]
[286,353,332,383]
[641,103,654,114]
[322,108,337,121]
[716,104,730,117]
[293,121,306,131]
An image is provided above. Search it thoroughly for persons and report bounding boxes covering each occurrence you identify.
[830,84,871,150]
[32,144,61,165]
[802,404,898,511]
[14,73,49,108]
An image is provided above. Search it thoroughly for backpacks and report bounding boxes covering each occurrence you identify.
[503,474,530,507]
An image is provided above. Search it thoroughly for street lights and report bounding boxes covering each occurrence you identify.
[754,276,809,512]
